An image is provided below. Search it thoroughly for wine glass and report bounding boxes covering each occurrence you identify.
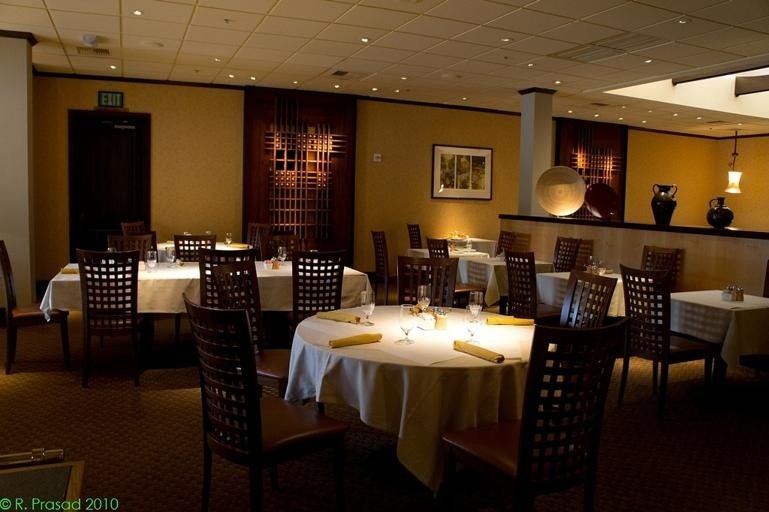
[469,292,482,323]
[395,304,417,344]
[360,290,375,326]
[278,246,286,266]
[417,285,431,320]
[225,232,233,248]
[165,246,175,267]
[146,250,156,272]
[464,305,481,344]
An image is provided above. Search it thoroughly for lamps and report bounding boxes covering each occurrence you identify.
[726,128,745,197]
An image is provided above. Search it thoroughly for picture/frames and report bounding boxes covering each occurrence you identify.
[431,142,496,203]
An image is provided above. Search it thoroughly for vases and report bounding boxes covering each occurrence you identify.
[706,196,735,229]
[650,181,681,226]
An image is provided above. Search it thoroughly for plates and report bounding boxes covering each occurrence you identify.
[585,182,619,220]
[535,165,586,217]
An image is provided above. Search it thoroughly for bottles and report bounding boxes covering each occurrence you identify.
[736,285,744,301]
